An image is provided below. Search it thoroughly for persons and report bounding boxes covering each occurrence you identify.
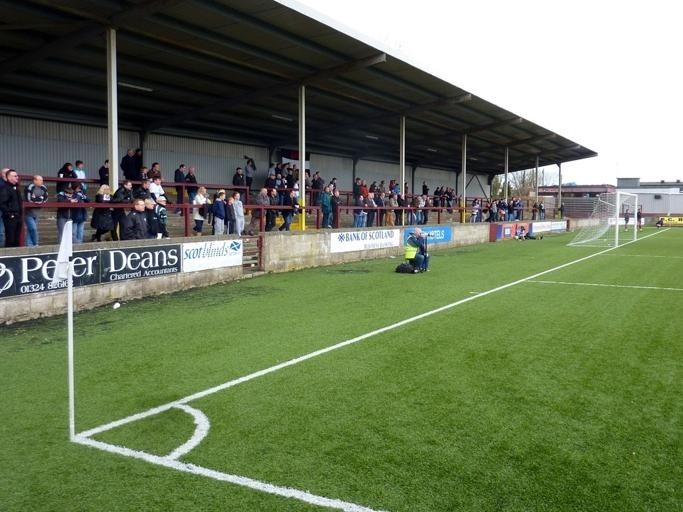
[233,192,245,236]
[557,201,566,220]
[99,160,109,187]
[233,167,247,215]
[243,170,252,202]
[121,199,149,241]
[402,226,429,275]
[1,169,23,248]
[73,160,87,190]
[132,179,152,201]
[154,196,168,232]
[192,186,211,236]
[111,180,134,242]
[185,167,199,213]
[519,226,525,239]
[23,174,49,248]
[174,164,189,213]
[56,162,78,195]
[1,168,11,248]
[532,201,539,220]
[654,217,663,229]
[322,177,343,229]
[148,176,166,202]
[539,201,545,220]
[143,196,169,239]
[213,189,221,203]
[71,183,90,244]
[212,192,225,236]
[636,208,642,232]
[241,163,324,236]
[90,184,113,242]
[352,177,432,228]
[226,196,239,234]
[141,167,147,180]
[147,162,171,200]
[218,189,227,205]
[57,188,78,245]
[120,148,143,191]
[432,186,455,214]
[469,197,524,223]
[623,208,630,232]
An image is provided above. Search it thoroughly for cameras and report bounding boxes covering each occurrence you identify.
[420,232,434,237]
